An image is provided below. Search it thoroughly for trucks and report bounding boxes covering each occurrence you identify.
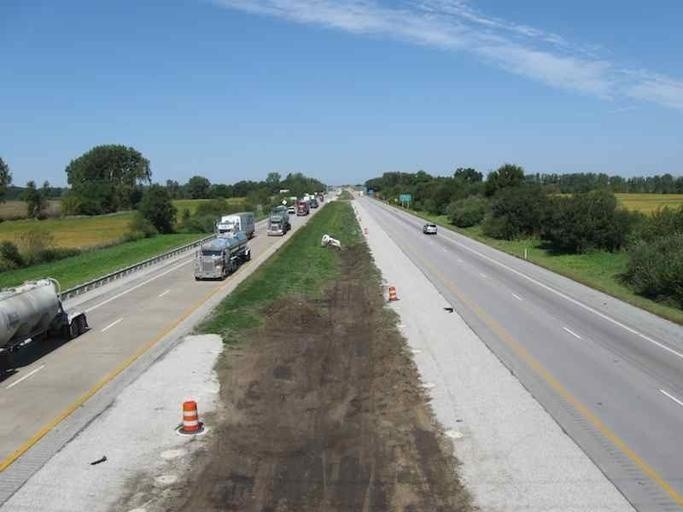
[266,190,326,237]
[216,211,254,241]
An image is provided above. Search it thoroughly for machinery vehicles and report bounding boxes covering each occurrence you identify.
[0,278,89,369]
[191,231,250,280]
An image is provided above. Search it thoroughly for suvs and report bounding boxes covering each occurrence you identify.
[423,223,437,234]
